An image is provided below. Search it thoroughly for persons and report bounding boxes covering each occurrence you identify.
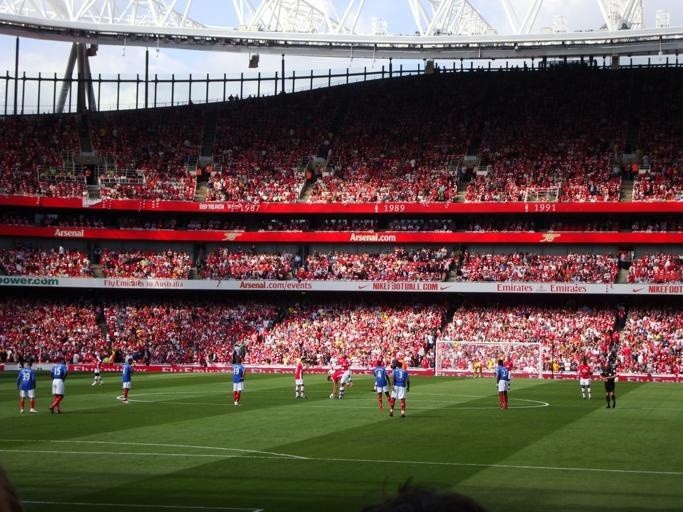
[496,359,510,409]
[15,359,39,414]
[371,361,392,412]
[0,90,683,376]
[232,358,244,406]
[389,360,411,418]
[115,357,134,405]
[576,358,594,399]
[325,369,353,399]
[292,357,307,399]
[599,361,619,408]
[47,359,69,414]
[88,358,104,386]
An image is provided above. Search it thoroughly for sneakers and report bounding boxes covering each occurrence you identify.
[121,400,128,404]
[116,395,125,400]
[612,402,616,408]
[295,394,306,398]
[605,406,611,408]
[401,410,405,417]
[370,389,376,393]
[29,407,40,413]
[55,409,61,414]
[49,404,54,414]
[233,401,238,406]
[583,398,591,400]
[389,408,394,416]
[20,408,24,415]
